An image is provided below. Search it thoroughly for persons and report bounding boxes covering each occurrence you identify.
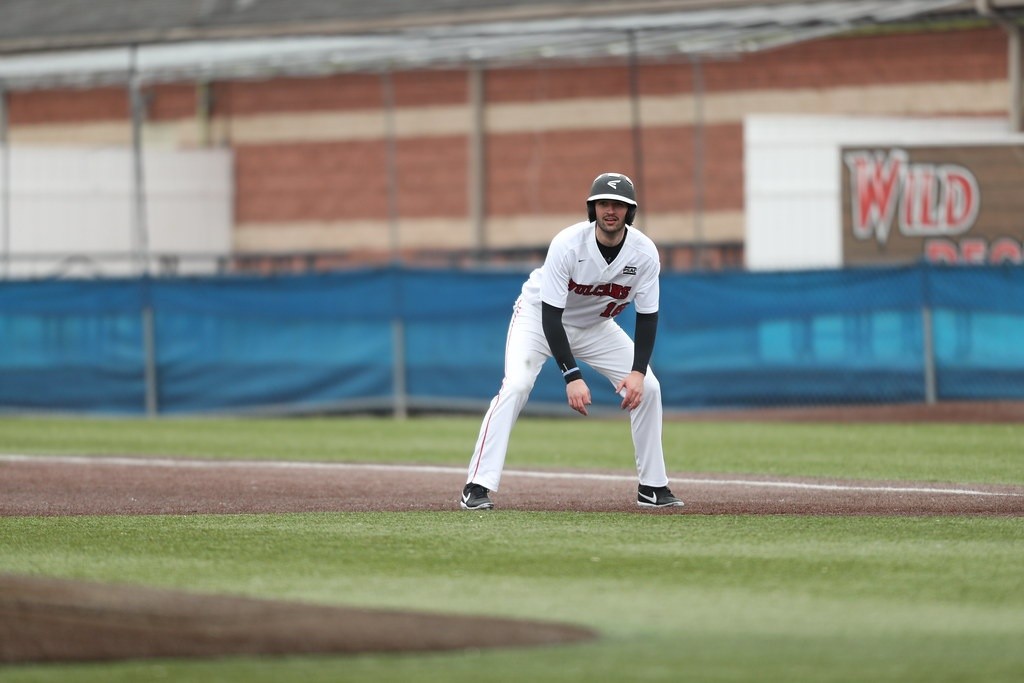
[459,171,685,511]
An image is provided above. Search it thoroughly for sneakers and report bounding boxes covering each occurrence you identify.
[460,482,494,510]
[637,483,686,508]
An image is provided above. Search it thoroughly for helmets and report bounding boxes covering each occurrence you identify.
[586,173,638,226]
